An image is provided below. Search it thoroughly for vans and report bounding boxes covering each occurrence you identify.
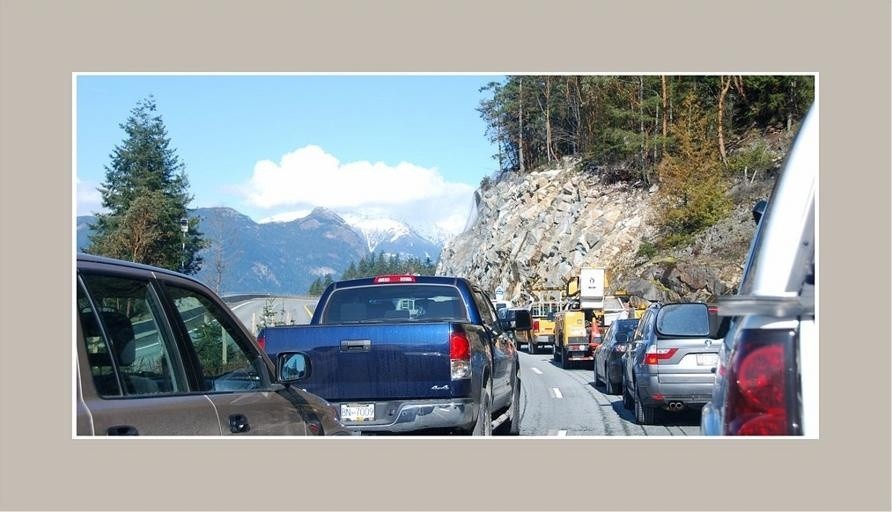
[701,99,819,435]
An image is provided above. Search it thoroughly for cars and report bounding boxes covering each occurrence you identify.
[77,254,355,438]
[593,302,718,424]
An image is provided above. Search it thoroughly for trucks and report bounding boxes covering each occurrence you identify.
[552,267,649,369]
